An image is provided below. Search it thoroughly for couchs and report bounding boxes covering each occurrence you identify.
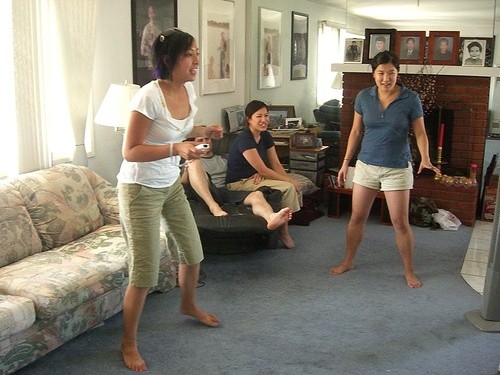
[0,164,177,375]
[179,133,282,250]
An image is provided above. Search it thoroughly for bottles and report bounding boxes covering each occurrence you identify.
[470,164,477,178]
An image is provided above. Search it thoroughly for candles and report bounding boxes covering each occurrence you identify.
[438,123,444,146]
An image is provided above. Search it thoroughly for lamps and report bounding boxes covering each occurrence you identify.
[92,79,141,136]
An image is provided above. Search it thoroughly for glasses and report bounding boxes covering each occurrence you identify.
[155,28,183,51]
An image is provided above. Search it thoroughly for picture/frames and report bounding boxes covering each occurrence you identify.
[395,30,426,64]
[294,132,316,150]
[198,0,236,96]
[462,39,486,66]
[343,38,364,64]
[291,11,309,81]
[363,28,395,63]
[265,106,295,129]
[428,31,459,65]
[131,0,178,88]
[257,7,283,89]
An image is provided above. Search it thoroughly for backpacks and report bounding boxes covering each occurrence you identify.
[409,195,440,229]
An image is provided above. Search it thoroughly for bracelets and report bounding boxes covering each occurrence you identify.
[344,157,351,163]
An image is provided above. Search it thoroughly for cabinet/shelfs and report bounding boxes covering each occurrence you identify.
[290,146,329,192]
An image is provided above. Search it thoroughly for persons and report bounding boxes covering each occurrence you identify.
[115,27,225,373]
[400,38,418,58]
[329,49,442,289]
[217,31,227,78]
[370,35,386,59]
[435,37,452,60]
[140,3,162,80]
[345,41,361,61]
[463,41,483,65]
[180,125,293,232]
[224,99,305,250]
